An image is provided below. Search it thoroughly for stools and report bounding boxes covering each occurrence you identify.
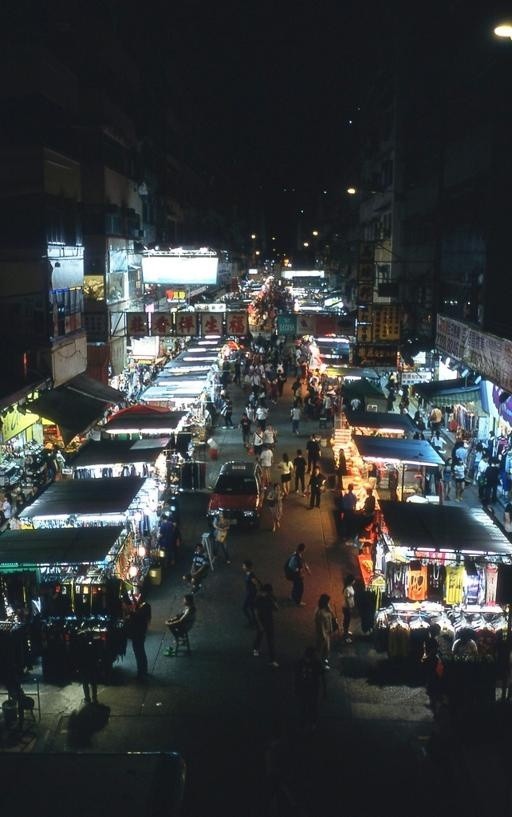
[174,632,192,657]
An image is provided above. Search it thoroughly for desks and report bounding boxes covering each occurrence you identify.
[329,437,396,598]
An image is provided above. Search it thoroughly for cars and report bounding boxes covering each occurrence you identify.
[206,460,268,529]
